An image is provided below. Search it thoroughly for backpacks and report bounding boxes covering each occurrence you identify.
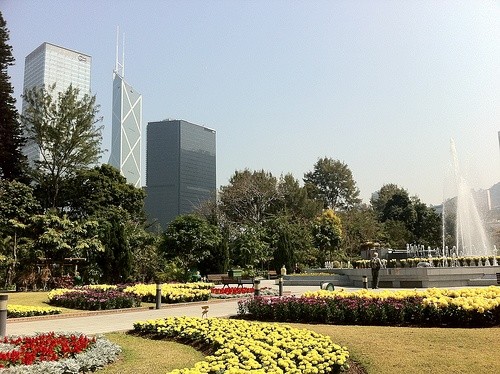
[371,257,378,269]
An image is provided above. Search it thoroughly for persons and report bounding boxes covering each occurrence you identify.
[281,265,286,277]
[370,252,383,289]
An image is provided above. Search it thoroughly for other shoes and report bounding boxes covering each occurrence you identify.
[372,287,379,289]
